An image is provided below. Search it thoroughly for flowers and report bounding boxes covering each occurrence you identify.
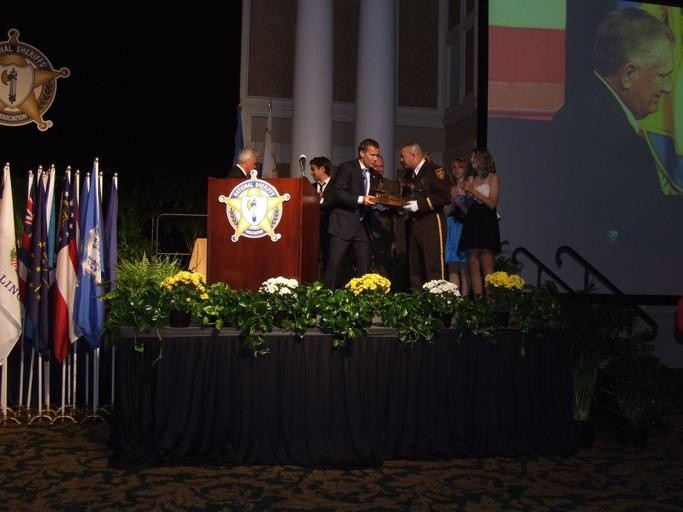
[161,271,209,309]
[259,275,299,301]
[423,278,461,313]
[484,270,526,311]
[345,272,392,300]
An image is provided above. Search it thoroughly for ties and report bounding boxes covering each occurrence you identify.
[318,182,325,192]
[361,169,368,219]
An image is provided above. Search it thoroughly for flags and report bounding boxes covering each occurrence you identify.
[0,158,119,368]
[261,111,279,178]
[230,113,246,168]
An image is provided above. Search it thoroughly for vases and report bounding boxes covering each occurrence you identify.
[362,310,374,327]
[169,309,191,327]
[432,314,453,327]
[492,311,511,328]
[272,310,288,326]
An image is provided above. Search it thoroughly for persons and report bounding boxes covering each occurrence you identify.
[226,148,258,176]
[505,6,683,295]
[310,137,501,293]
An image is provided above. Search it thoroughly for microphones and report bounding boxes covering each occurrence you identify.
[299,154,307,176]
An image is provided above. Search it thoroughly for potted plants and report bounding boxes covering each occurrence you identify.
[568,342,597,449]
[612,356,671,447]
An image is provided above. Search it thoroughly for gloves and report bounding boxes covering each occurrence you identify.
[403,200,419,212]
